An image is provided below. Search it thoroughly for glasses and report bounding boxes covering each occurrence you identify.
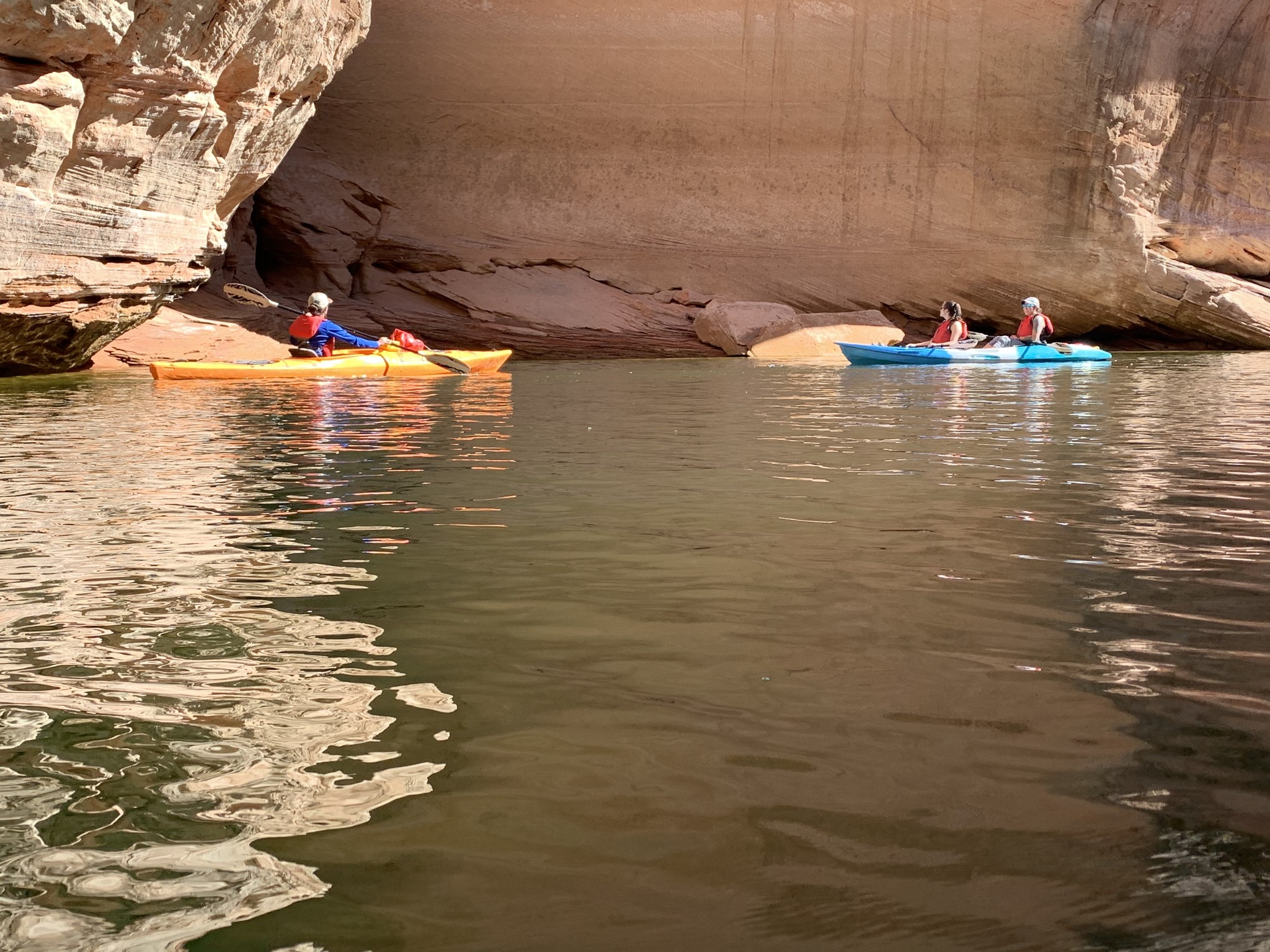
[1023,304,1034,308]
[940,307,946,310]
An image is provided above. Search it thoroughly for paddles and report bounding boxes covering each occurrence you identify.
[967,330,1073,355]
[871,339,978,348]
[223,283,470,374]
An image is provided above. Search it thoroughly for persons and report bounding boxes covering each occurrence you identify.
[288,292,393,357]
[872,301,963,347]
[982,297,1046,348]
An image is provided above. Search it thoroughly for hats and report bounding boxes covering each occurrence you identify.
[308,292,333,309]
[1021,297,1040,307]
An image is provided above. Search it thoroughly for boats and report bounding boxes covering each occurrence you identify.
[833,342,1112,365]
[149,348,513,381]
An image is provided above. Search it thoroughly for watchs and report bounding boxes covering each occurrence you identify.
[1016,336,1020,340]
